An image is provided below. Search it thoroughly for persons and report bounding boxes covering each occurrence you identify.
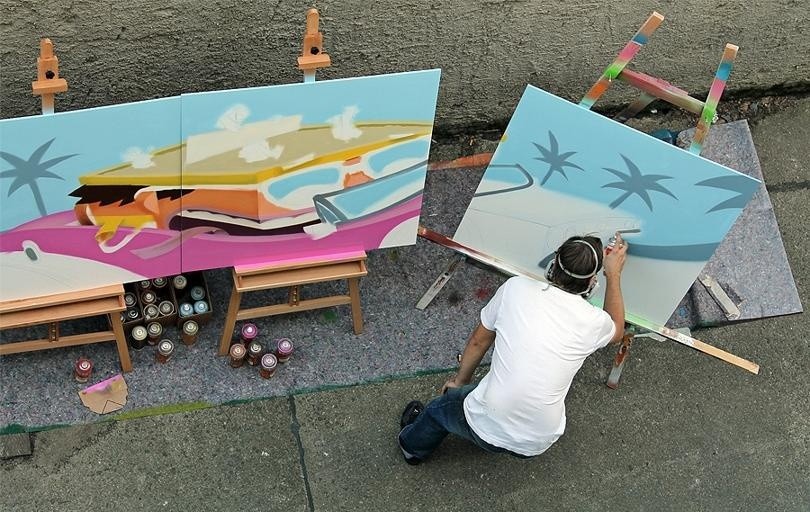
[397,231,628,466]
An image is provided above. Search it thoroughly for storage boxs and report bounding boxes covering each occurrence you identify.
[169,270,213,329]
[107,284,144,332]
[135,276,178,326]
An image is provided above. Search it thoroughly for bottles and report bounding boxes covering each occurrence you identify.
[603,236,622,277]
[74,358,93,383]
[118,276,207,362]
[228,323,293,379]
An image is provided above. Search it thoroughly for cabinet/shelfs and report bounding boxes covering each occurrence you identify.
[219,251,368,355]
[0,285,132,373]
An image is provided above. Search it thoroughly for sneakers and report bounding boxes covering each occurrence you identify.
[401,400,424,465]
